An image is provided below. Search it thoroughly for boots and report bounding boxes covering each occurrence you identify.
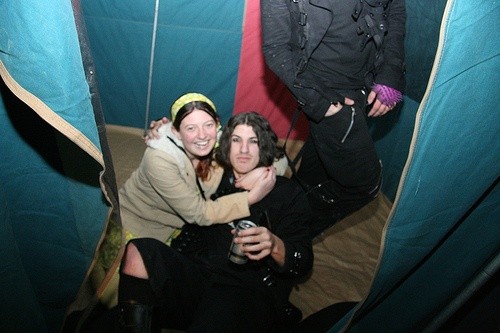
[118,304,153,333]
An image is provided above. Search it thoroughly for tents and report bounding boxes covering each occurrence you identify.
[0,0,499,333]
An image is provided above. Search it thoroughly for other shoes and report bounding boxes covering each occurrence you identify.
[295,239,313,284]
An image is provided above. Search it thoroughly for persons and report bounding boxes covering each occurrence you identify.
[143,111,313,333]
[115,94,276,333]
[260,0,407,239]
